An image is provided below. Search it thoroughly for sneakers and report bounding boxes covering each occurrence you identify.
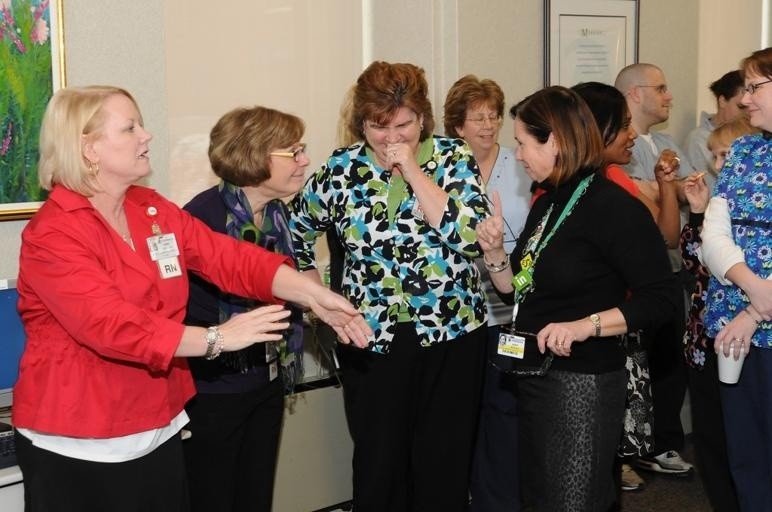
[635,448,695,480]
[620,464,646,491]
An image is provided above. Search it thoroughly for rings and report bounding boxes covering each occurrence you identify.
[394,151,396,155]
[557,342,563,345]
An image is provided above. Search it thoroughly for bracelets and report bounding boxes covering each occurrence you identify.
[207,326,223,361]
[484,254,511,272]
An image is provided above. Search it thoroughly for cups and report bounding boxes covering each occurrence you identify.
[718,343,745,385]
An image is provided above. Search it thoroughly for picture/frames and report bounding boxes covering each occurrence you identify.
[0,0,65,221]
[543,0,641,91]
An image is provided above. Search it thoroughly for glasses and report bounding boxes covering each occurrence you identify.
[742,79,772,95]
[465,114,502,124]
[637,85,667,94]
[270,143,306,161]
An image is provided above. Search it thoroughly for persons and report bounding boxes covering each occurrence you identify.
[445,73,534,511]
[477,86,674,510]
[574,63,693,491]
[285,60,490,510]
[680,48,772,511]
[9,84,374,512]
[179,108,311,512]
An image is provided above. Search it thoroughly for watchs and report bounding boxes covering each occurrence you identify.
[204,325,218,360]
